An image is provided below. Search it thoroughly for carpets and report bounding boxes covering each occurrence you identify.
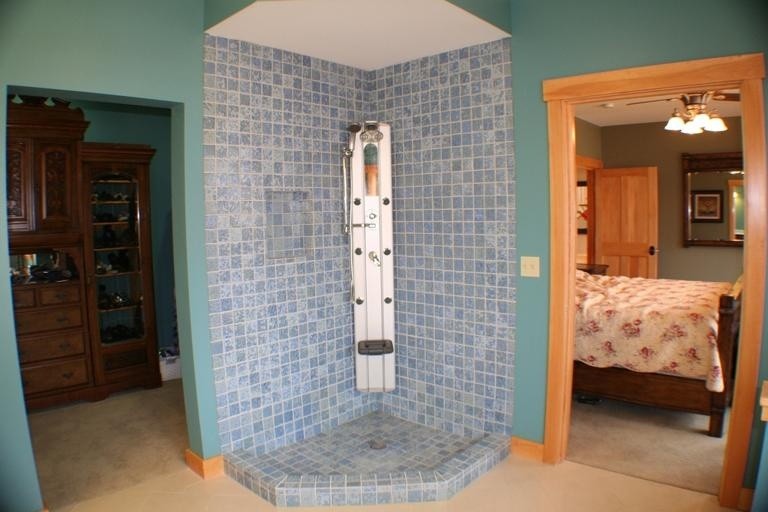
[27,381,198,511]
[567,398,732,497]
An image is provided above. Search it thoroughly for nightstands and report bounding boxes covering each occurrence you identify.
[577,262,611,278]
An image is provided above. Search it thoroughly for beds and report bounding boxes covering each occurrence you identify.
[573,271,749,438]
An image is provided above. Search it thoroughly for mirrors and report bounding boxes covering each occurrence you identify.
[679,149,744,247]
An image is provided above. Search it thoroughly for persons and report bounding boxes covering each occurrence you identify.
[23,255,32,283]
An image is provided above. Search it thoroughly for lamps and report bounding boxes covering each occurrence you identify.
[664,102,729,135]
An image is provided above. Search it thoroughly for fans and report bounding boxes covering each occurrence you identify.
[625,90,741,107]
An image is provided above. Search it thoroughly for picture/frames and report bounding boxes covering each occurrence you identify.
[691,189,725,222]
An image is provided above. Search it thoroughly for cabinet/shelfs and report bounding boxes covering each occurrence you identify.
[4,97,162,415]
